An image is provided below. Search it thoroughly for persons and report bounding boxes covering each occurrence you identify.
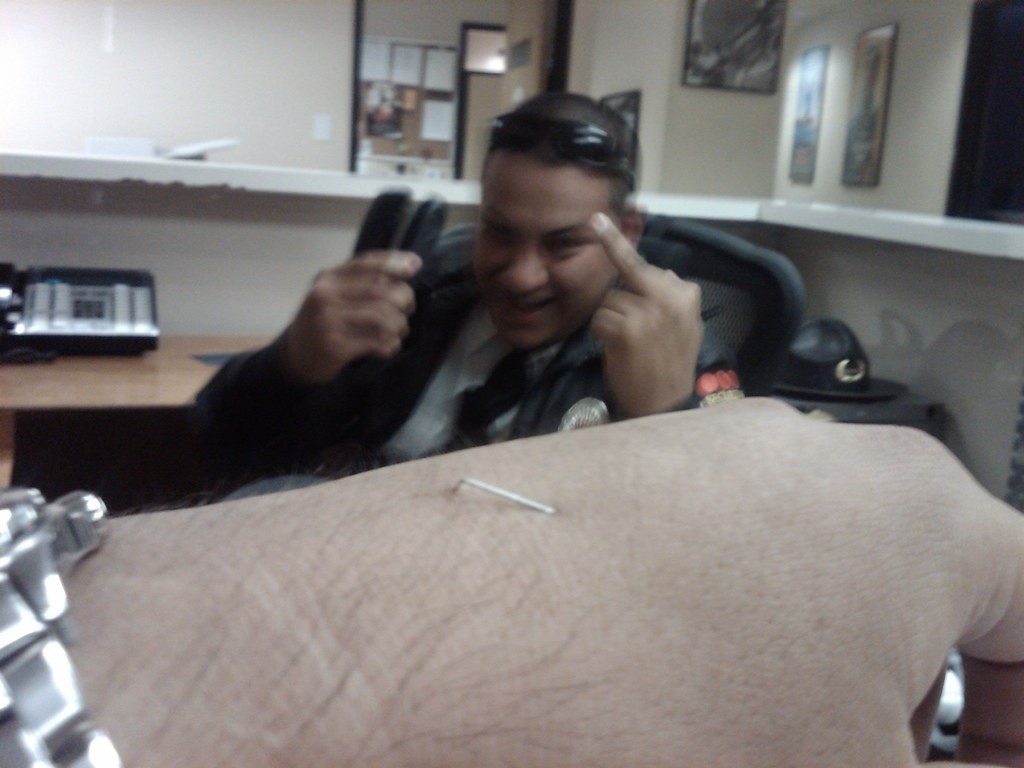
[195,93,747,493]
[0,395,1024,768]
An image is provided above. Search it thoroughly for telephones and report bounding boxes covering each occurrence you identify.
[1,261,160,360]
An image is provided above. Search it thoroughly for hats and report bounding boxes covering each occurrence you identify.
[771,317,909,402]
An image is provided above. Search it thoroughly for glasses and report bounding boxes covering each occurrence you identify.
[490,111,635,189]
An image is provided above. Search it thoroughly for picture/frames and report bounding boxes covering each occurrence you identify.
[789,42,829,182]
[600,89,641,191]
[840,21,899,184]
[681,0,787,95]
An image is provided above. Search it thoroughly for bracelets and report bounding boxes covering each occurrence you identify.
[0,487,122,767]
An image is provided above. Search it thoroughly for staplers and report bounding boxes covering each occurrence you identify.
[343,190,447,392]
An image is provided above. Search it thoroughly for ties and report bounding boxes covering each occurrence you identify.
[446,351,526,447]
